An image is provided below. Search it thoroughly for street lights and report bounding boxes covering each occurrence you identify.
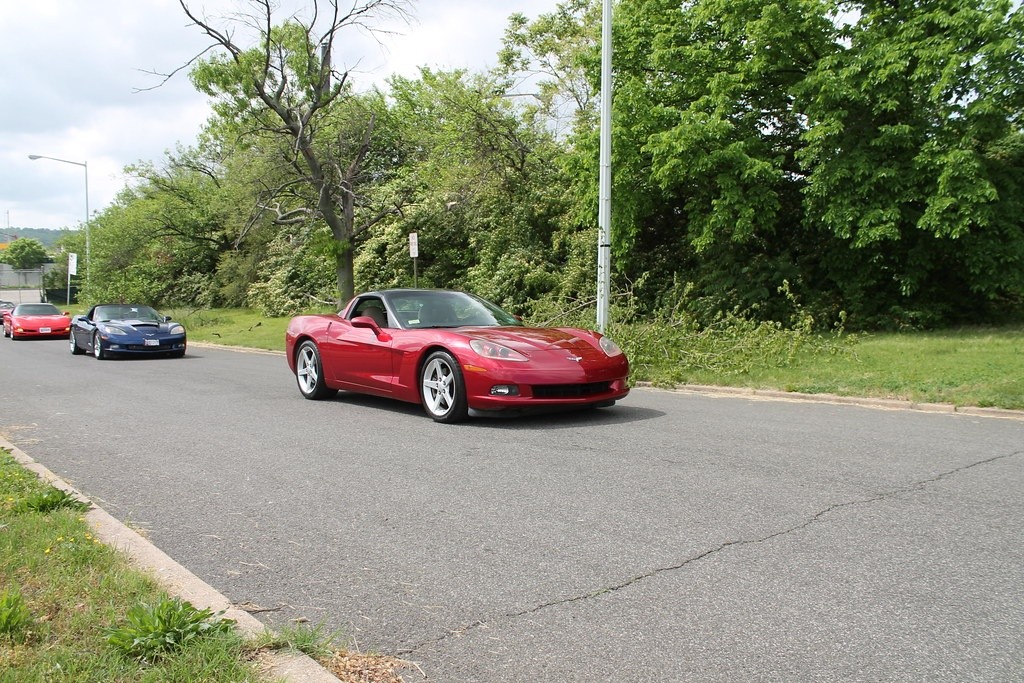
[27,153,91,306]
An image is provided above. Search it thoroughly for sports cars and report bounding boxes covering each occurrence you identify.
[285,289,633,424]
[2,302,72,340]
[68,303,187,360]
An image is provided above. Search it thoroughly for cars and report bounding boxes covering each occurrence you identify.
[0,300,17,322]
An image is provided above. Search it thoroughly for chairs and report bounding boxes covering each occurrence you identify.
[363,306,386,327]
[419,303,458,323]
